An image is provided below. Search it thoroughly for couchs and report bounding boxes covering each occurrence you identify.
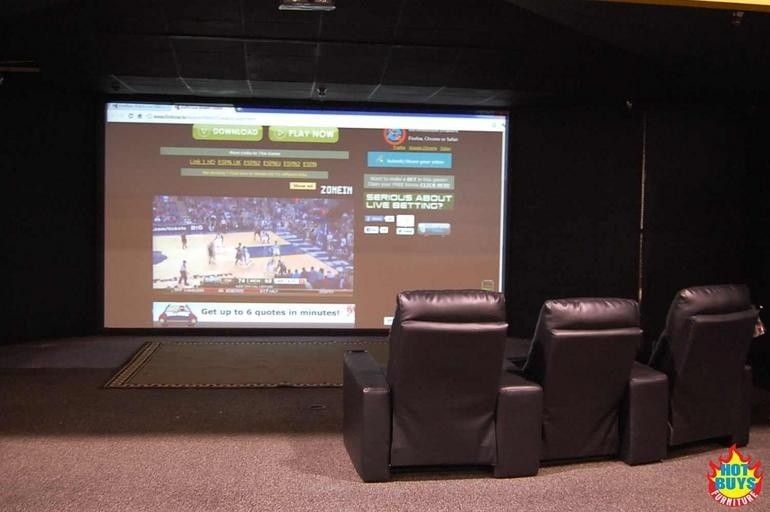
[340,276,760,485]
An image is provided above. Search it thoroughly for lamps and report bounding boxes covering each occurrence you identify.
[277,0,337,11]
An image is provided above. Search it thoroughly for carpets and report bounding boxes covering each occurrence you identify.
[102,339,388,391]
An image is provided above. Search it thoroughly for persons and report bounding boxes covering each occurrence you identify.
[153,196,354,291]
